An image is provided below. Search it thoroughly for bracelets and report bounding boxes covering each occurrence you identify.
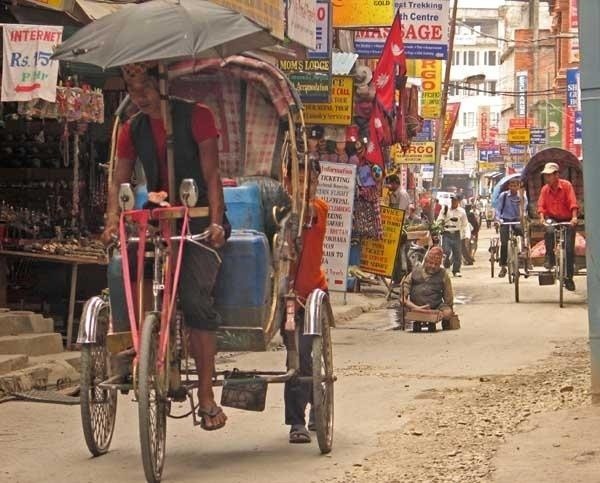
[212,222,225,236]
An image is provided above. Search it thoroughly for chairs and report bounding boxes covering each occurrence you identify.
[528,199,585,232]
[133,181,266,234]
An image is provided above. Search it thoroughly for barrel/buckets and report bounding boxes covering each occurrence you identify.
[346,247,361,291]
[212,227,270,307]
[221,177,266,231]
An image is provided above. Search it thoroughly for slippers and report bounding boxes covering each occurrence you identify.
[197,407,226,430]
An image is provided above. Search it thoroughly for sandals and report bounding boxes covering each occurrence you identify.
[288,424,311,443]
[308,408,317,431]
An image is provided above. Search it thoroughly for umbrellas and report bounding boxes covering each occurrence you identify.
[46,0,286,268]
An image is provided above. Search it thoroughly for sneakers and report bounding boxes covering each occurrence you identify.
[498,268,507,278]
[564,278,576,291]
[544,256,556,268]
[454,272,463,277]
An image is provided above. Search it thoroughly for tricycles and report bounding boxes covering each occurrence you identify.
[75,49,337,483]
[488,146,585,308]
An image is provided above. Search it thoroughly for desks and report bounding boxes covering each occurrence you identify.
[405,230,433,249]
[0,249,110,351]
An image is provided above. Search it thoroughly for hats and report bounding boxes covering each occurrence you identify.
[540,162,560,174]
[450,195,459,200]
[428,247,443,261]
[120,60,158,78]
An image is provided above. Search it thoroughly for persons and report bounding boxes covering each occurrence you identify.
[385,162,578,330]
[98,58,227,431]
[277,157,329,441]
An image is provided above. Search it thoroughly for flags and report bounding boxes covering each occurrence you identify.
[0,23,64,103]
